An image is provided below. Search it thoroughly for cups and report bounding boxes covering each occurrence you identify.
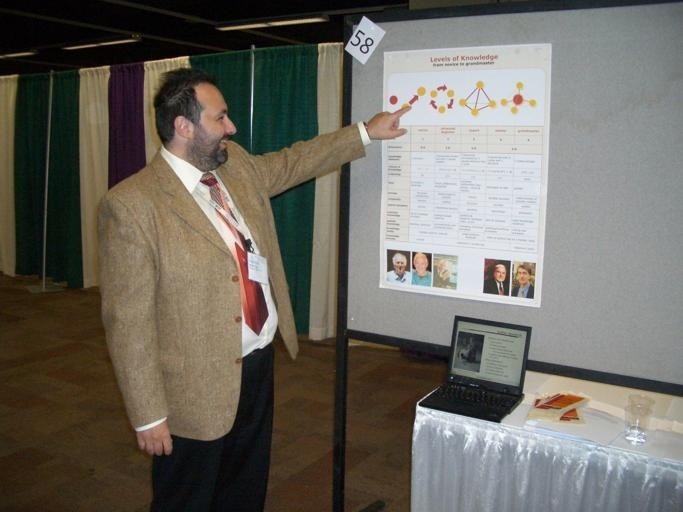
[625,394,652,445]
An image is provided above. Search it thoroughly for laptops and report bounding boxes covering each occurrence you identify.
[419,315,532,423]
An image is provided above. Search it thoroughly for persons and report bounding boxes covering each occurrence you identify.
[410,253,431,287]
[431,258,456,290]
[96,67,411,512]
[483,264,508,295]
[385,252,410,285]
[511,264,534,299]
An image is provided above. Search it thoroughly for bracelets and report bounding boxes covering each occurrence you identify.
[363,122,368,135]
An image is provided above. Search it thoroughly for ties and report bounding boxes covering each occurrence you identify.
[499,283,503,294]
[200,173,268,337]
[518,291,524,297]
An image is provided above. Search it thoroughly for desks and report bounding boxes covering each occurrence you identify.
[410,370,683,512]
